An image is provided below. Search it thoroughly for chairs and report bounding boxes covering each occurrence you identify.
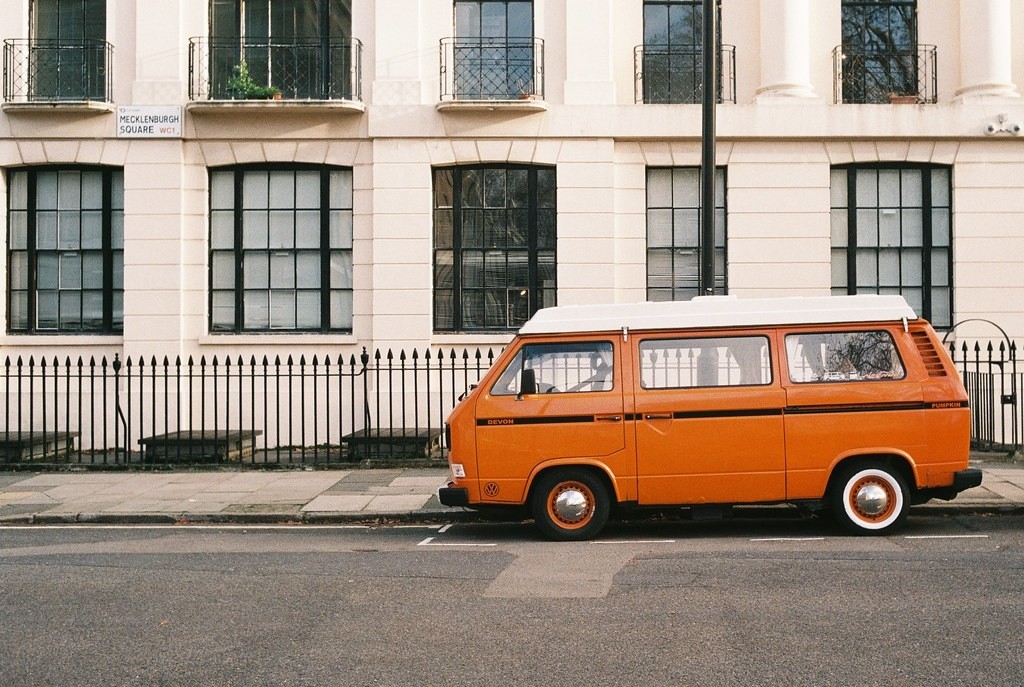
[592,362,606,393]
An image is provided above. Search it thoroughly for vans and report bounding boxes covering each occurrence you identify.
[436,293,984,542]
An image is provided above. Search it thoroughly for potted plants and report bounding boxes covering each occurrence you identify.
[518,79,537,102]
[227,61,282,100]
[888,88,917,103]
